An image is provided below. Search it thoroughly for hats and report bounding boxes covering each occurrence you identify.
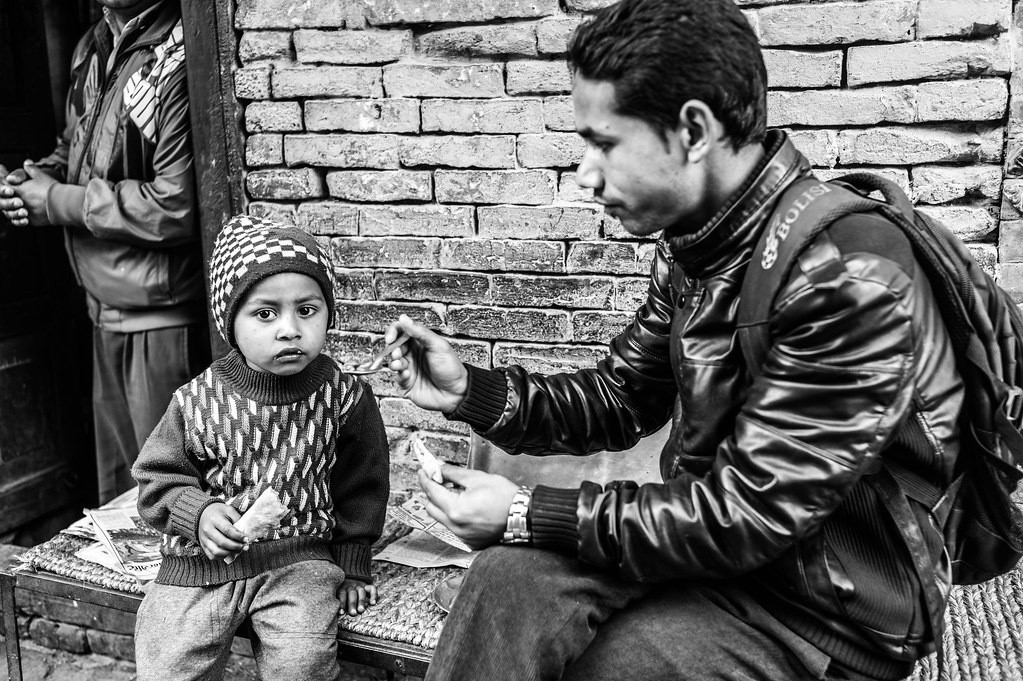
[207,214,335,350]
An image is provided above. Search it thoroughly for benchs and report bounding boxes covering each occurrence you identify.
[1,479,476,680]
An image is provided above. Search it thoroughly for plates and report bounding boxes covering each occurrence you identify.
[432,575,467,615]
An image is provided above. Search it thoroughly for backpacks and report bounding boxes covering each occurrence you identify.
[669,170,1023,585]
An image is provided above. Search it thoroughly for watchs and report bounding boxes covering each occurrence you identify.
[502,482,532,546]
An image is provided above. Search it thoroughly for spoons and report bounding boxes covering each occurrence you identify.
[340,321,424,374]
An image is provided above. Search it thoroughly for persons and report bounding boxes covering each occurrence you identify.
[381,0,962,681]
[129,206,393,681]
[0,0,203,502]
